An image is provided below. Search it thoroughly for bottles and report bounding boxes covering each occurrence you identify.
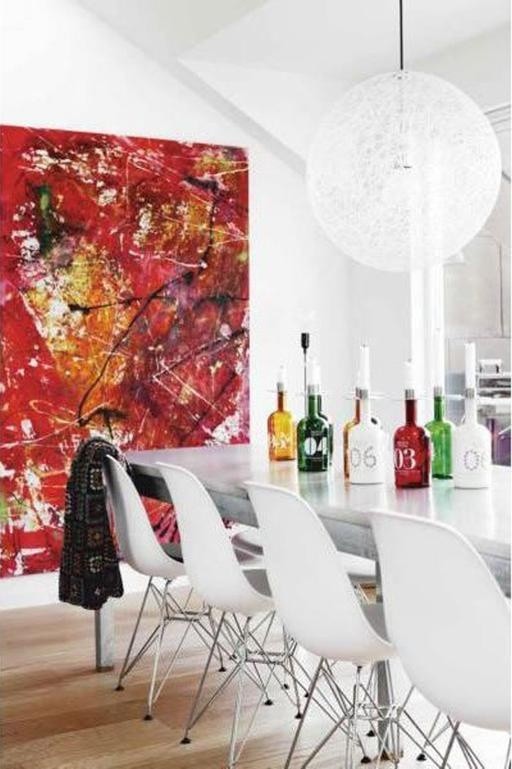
[452,389,491,488]
[268,381,296,460]
[296,385,334,472]
[343,385,390,486]
[394,389,431,486]
[422,386,452,480]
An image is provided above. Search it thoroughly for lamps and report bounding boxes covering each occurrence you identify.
[305,0,495,267]
